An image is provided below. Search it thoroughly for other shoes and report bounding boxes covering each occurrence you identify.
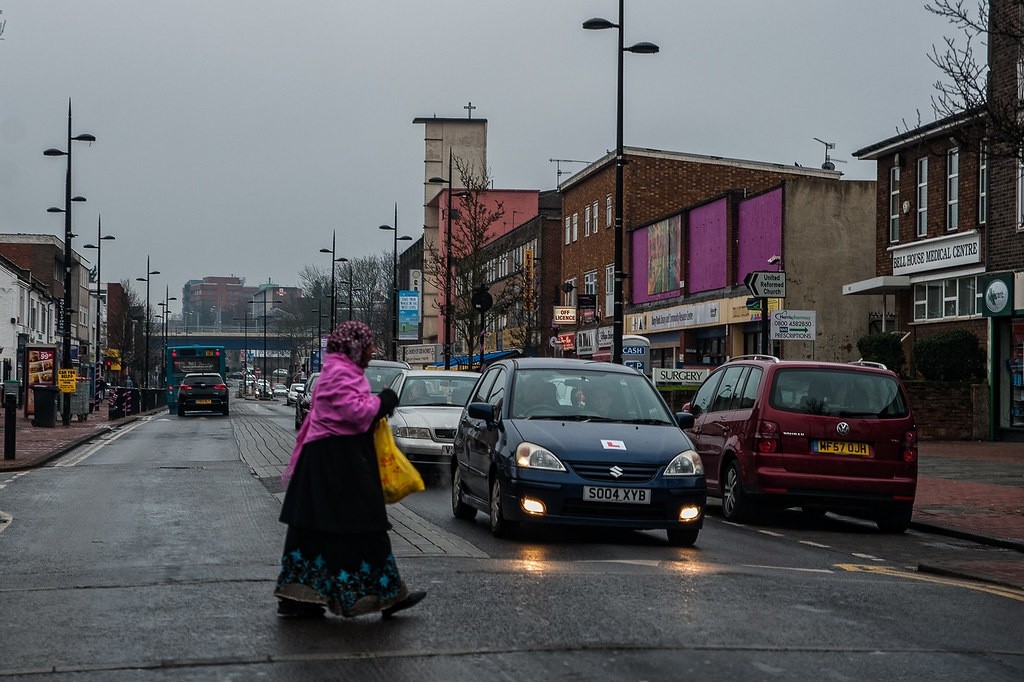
[277,599,327,617]
[382,590,427,618]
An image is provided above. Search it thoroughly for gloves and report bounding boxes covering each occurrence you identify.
[375,388,400,416]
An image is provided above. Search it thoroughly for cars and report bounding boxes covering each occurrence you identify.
[449,356,708,547]
[286,383,305,406]
[177,372,229,417]
[294,372,321,430]
[364,359,412,396]
[382,369,485,473]
[227,367,290,397]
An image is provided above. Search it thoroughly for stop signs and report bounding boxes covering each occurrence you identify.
[254,368,262,376]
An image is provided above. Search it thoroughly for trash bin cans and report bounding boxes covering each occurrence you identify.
[31,387,59,428]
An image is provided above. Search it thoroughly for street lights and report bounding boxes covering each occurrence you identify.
[428,145,453,371]
[41,95,97,428]
[248,290,283,401]
[83,213,115,364]
[319,228,349,334]
[154,283,178,368]
[232,309,261,396]
[135,254,160,389]
[581,0,661,367]
[377,201,413,363]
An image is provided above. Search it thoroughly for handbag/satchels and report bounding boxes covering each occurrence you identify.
[374,417,425,504]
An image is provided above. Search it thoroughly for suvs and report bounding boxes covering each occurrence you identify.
[681,351,919,534]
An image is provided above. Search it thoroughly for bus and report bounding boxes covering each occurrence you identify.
[166,343,227,416]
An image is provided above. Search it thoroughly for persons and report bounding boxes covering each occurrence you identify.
[519,376,626,417]
[407,380,426,401]
[99,376,133,403]
[272,321,428,620]
